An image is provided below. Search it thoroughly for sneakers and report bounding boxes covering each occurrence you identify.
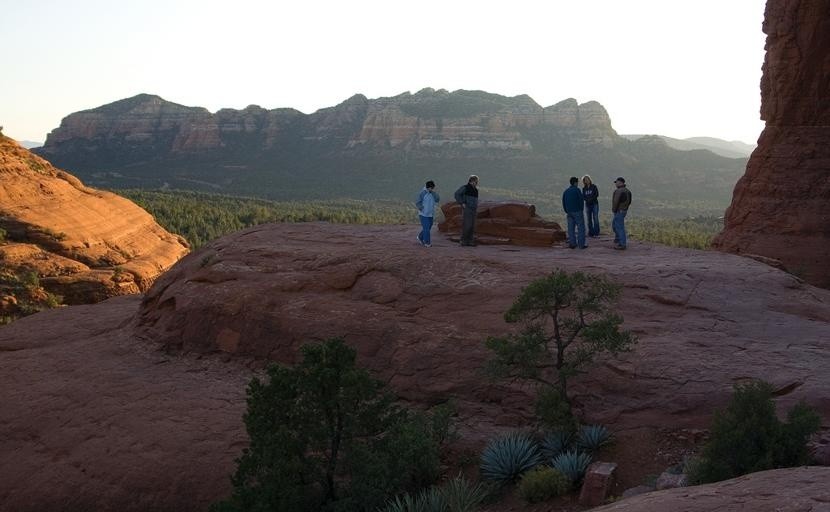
[416,235,423,245]
[424,244,432,248]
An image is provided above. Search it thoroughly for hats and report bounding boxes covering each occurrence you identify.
[426,181,435,188]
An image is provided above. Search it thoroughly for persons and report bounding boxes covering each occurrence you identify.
[455,174,479,247]
[610,176,632,250]
[562,176,588,249]
[582,175,600,236]
[415,179,440,248]
